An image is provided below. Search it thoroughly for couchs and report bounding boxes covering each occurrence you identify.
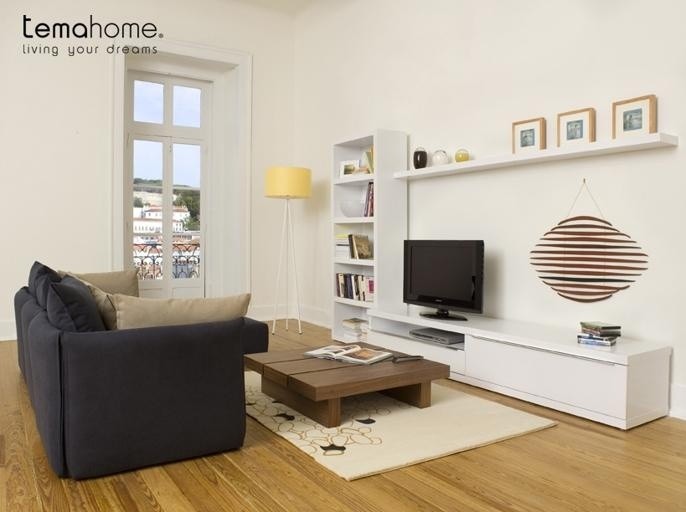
[11,286,268,479]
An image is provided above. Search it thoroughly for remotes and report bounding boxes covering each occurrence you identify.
[392,356,423,363]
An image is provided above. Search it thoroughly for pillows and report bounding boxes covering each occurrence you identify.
[29,261,251,332]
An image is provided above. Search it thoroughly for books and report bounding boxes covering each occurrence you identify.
[304,345,393,365]
[577,321,621,346]
[334,182,374,301]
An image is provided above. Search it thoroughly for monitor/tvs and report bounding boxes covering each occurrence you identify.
[404,239,484,321]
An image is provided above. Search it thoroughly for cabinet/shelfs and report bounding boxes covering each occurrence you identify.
[329,127,408,345]
[362,310,674,432]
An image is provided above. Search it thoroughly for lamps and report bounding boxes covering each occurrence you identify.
[265,165,311,335]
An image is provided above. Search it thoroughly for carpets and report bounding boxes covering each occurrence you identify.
[240,370,557,482]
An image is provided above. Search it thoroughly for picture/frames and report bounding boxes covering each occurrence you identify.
[510,117,545,154]
[557,107,597,147]
[612,94,656,141]
[340,159,360,178]
